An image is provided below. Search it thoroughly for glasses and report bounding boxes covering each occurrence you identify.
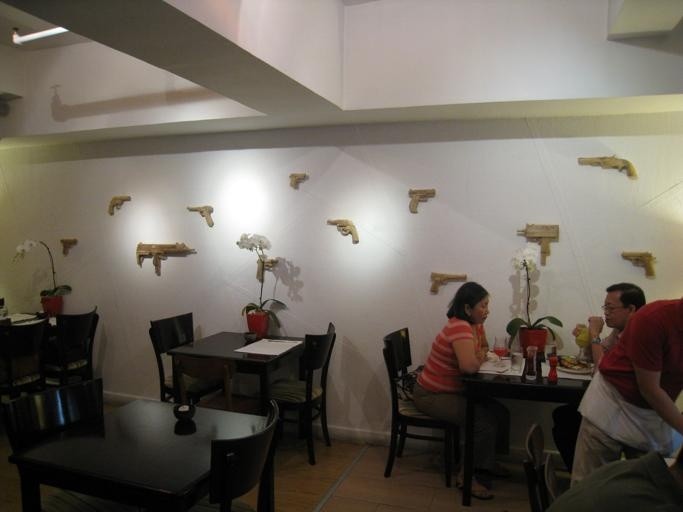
[600,303,632,310]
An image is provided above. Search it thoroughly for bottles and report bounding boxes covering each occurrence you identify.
[524,345,538,381]
[547,353,557,384]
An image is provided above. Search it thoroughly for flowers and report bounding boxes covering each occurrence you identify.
[507,241,563,345]
[235,232,285,329]
[12,239,73,299]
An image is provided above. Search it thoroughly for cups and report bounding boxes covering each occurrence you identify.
[242,332,256,343]
[510,352,522,374]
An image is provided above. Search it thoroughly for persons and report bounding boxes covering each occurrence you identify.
[411,280,512,501]
[546,447,682,511]
[569,297,683,487]
[573,282,643,366]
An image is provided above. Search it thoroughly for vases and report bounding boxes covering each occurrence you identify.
[40,295,64,314]
[519,327,546,357]
[243,311,269,337]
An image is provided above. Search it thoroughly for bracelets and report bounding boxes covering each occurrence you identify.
[590,336,600,343]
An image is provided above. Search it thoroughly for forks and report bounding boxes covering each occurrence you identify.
[476,368,508,374]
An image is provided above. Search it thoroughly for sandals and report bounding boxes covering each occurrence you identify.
[456,466,513,499]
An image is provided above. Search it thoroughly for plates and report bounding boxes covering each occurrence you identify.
[556,357,596,374]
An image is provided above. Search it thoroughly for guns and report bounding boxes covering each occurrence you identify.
[136,242,196,276]
[620,251,656,278]
[60,238,77,256]
[408,187,435,212]
[256,259,278,280]
[187,205,214,227]
[326,218,359,244]
[108,195,131,215]
[430,271,467,294]
[517,222,559,265]
[289,173,306,188]
[578,155,637,178]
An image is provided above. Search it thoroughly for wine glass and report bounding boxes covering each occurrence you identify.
[575,322,589,363]
[493,335,507,368]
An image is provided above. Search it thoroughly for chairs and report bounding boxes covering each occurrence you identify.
[190,400,281,511]
[543,453,561,499]
[380,325,462,488]
[523,422,550,511]
[267,321,337,465]
[45,305,101,389]
[38,377,104,428]
[0,387,62,454]
[148,310,230,405]
[0,317,50,393]
